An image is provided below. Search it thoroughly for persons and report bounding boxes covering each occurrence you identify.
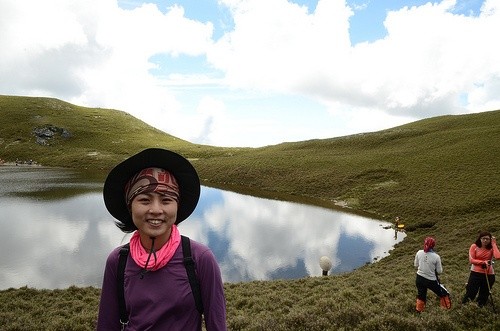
[413,237,452,315]
[97,148,227,331]
[462,232,500,308]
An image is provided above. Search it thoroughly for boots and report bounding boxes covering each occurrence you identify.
[440,294,451,309]
[415,297,426,313]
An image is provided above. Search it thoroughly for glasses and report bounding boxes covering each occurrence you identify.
[481,237,490,240]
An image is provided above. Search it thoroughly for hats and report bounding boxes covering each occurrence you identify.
[104,148,199,230]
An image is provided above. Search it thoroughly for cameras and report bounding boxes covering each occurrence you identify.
[489,260,495,265]
